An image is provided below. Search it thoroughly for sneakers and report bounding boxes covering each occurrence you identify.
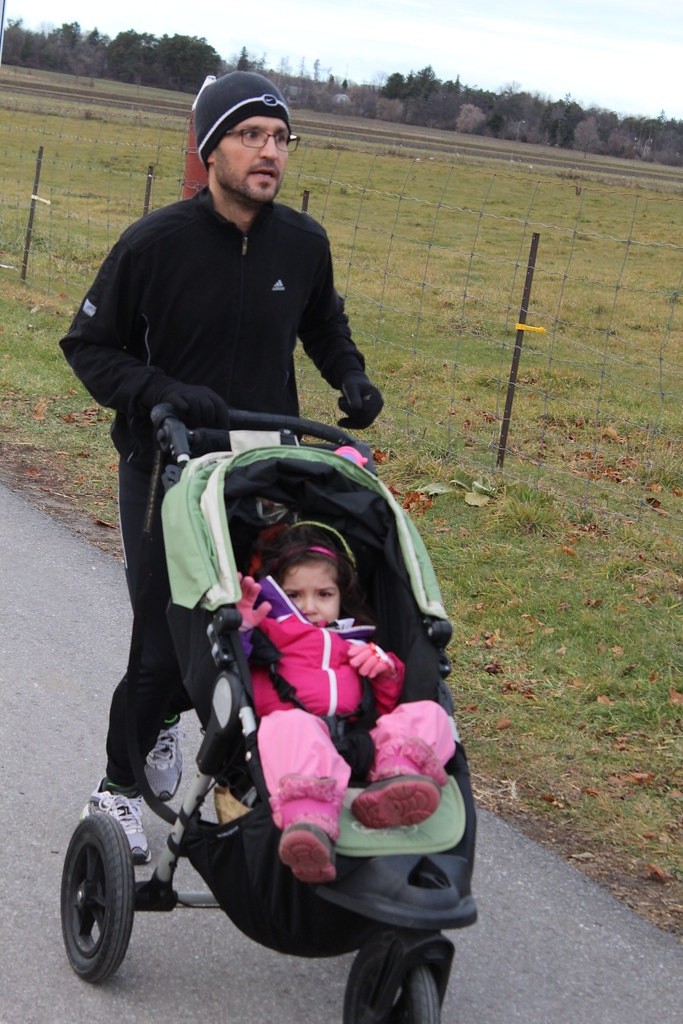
[77,776,152,865]
[143,713,183,802]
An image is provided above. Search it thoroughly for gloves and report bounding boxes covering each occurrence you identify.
[236,572,271,632]
[346,642,395,679]
[155,382,228,430]
[338,374,383,430]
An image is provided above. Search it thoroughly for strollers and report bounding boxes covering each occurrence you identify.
[61,403,478,1023]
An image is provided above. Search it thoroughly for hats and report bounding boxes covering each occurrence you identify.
[195,71,291,173]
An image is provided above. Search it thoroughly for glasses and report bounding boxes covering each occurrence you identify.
[227,129,300,153]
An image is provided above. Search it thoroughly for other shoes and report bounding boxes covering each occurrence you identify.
[279,823,335,882]
[351,775,442,829]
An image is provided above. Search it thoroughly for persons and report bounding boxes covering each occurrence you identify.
[229,520,456,883]
[58,70,383,865]
